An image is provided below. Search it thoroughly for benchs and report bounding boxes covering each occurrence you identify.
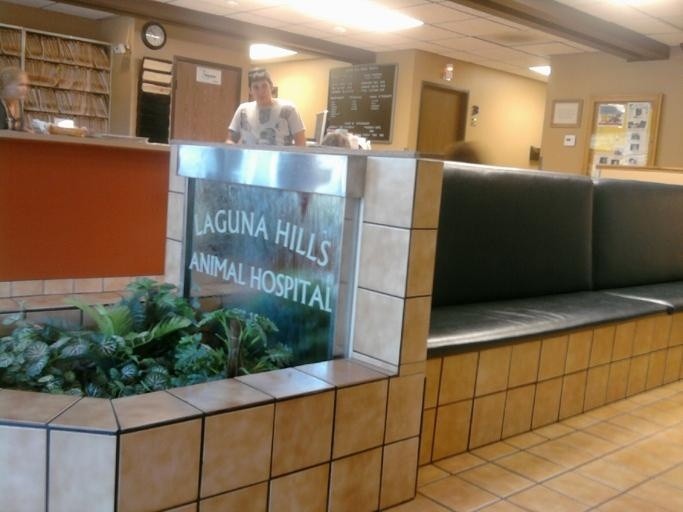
[419,156,681,468]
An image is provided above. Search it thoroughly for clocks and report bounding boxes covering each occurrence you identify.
[142,21,167,51]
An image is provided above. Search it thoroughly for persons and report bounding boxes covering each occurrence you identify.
[224,67,305,147]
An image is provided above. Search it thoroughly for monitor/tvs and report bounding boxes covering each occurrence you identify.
[315,111,329,144]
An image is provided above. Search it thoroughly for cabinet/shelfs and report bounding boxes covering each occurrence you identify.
[0,22,115,137]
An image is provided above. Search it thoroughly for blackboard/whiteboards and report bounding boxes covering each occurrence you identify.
[325,63,399,144]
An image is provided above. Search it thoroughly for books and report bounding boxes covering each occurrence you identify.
[1,26,112,134]
